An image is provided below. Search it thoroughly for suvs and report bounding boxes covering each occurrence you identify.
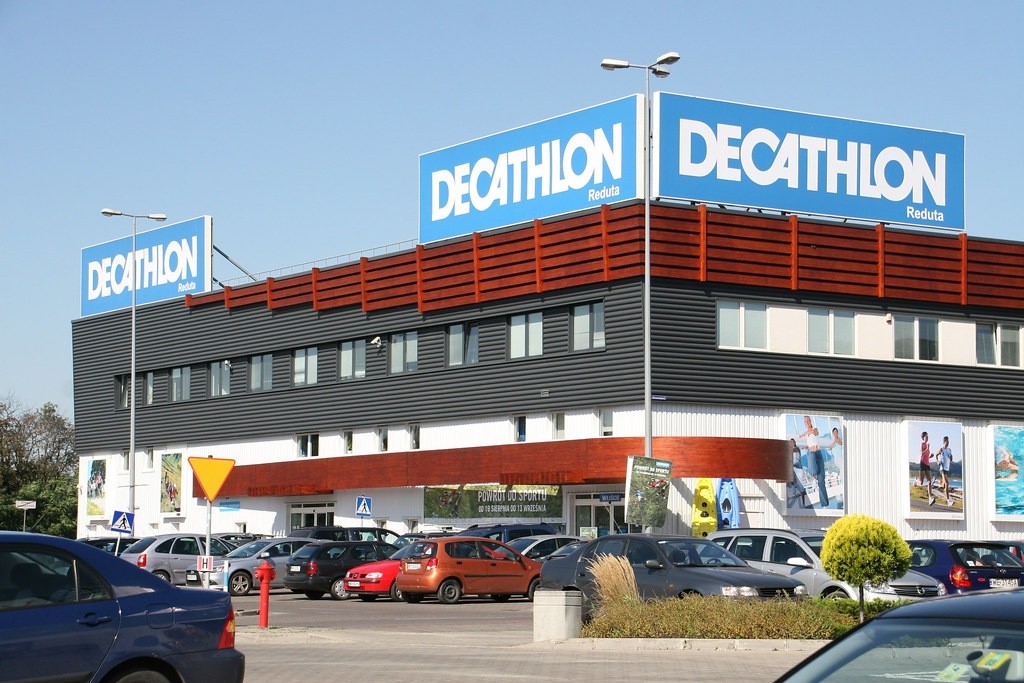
[682,526,949,611]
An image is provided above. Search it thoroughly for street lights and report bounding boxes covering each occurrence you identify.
[600,51,682,537]
[100,208,168,541]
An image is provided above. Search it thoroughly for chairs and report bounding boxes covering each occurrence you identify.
[668,551,686,563]
[11,563,48,598]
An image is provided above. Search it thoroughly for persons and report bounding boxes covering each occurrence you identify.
[912,431,936,506]
[165,471,178,501]
[90,473,104,499]
[829,428,841,473]
[797,415,830,506]
[789,438,807,509]
[936,435,955,508]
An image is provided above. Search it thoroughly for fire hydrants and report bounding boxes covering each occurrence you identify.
[254,561,277,628]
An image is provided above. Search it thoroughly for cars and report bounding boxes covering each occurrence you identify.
[76,518,1024,609]
[0,530,246,683]
[343,543,413,602]
[538,532,809,606]
[184,536,346,597]
[281,540,403,601]
[771,586,1024,683]
[395,535,544,605]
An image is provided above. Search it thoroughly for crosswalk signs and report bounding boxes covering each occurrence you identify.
[111,511,135,534]
[355,496,373,518]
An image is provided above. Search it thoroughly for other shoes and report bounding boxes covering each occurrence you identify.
[947,500,955,507]
[929,498,935,506]
[914,477,917,487]
